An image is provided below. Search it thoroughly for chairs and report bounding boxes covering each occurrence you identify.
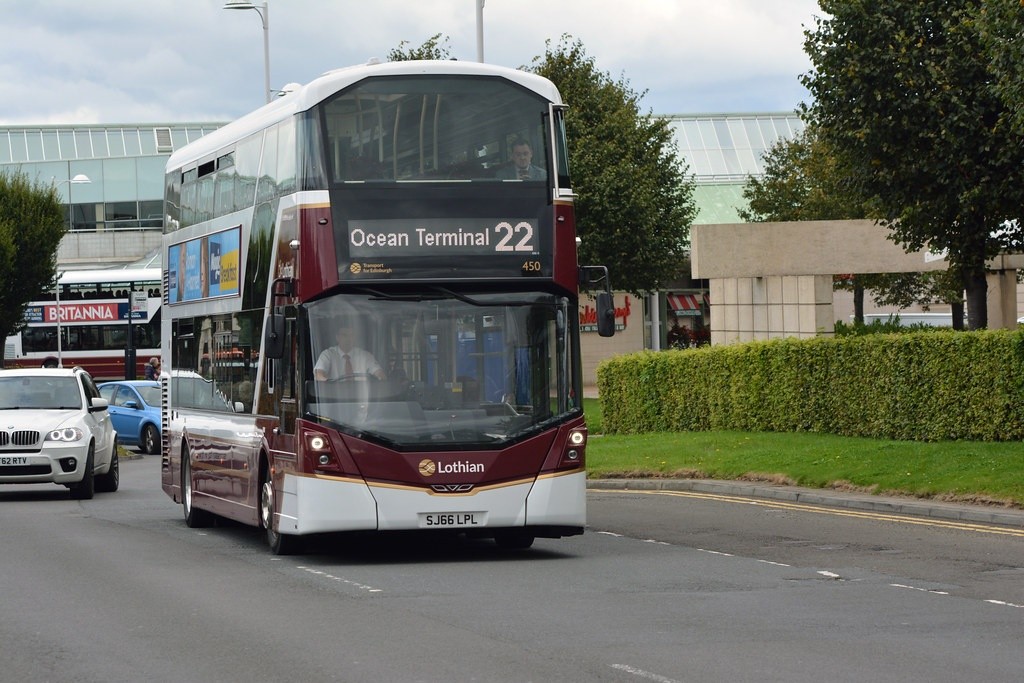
[38,288,161,301]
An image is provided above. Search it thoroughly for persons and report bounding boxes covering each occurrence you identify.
[493,139,547,181]
[143,355,160,381]
[312,321,387,382]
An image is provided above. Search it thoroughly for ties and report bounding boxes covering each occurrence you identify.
[343,354,355,382]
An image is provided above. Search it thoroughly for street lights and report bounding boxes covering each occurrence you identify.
[55,174,91,368]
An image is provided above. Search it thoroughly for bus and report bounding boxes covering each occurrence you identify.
[3,268,161,379]
[161,57,615,554]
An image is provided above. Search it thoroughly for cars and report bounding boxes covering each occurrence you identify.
[97,380,162,455]
[161,370,269,462]
[0,366,120,499]
